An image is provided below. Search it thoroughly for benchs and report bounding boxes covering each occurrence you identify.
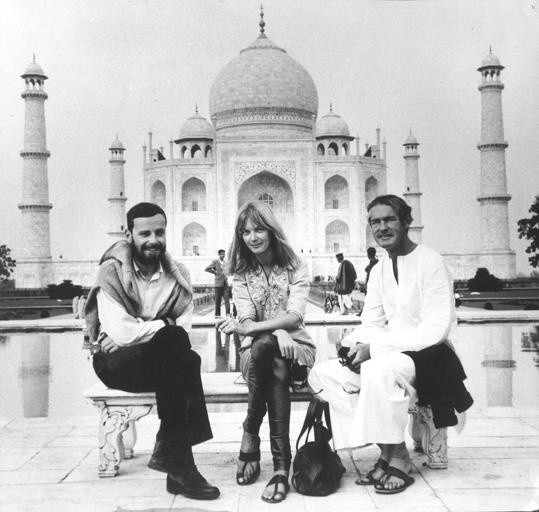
[86,370,451,477]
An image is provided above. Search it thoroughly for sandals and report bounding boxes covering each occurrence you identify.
[355,459,415,494]
[261,475,289,504]
[236,433,261,485]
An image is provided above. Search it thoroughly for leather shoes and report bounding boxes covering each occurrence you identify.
[148,441,219,500]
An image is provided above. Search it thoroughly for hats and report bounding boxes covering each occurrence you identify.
[336,253,343,259]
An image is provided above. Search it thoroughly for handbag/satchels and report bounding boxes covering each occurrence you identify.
[292,401,344,496]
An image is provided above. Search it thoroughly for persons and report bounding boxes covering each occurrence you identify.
[204,249,233,320]
[213,200,316,504]
[339,195,473,495]
[333,252,360,315]
[91,202,220,500]
[356,246,380,317]
